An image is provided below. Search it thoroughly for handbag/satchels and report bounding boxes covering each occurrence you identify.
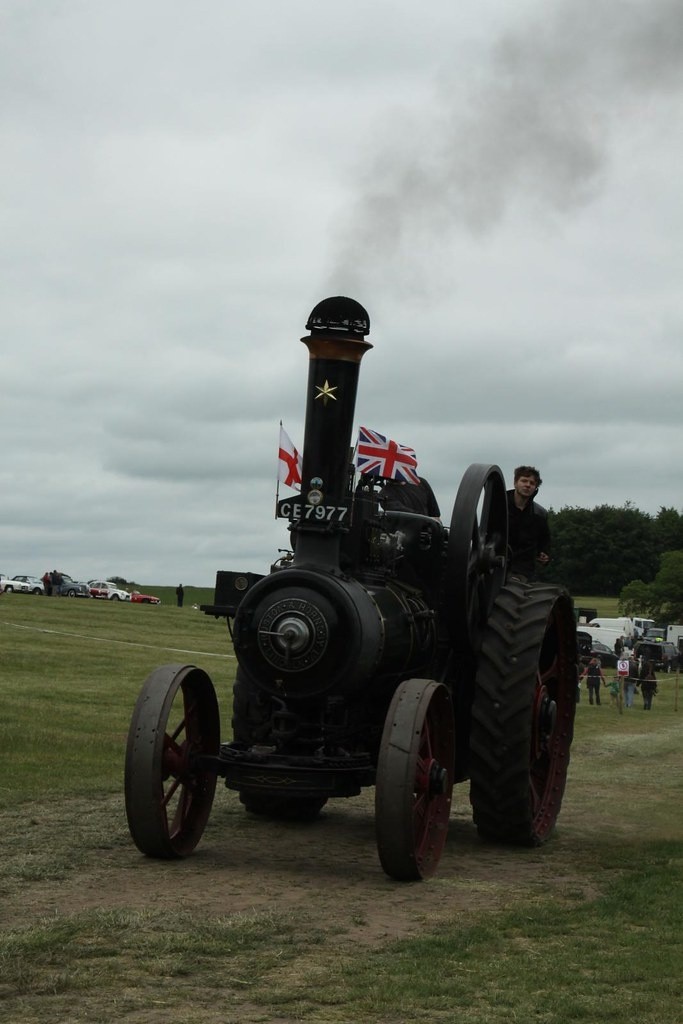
[645,670,655,684]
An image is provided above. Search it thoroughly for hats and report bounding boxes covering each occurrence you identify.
[627,656,636,663]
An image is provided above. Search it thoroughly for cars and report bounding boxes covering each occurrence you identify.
[129,590,162,605]
[86,580,131,602]
[588,639,621,669]
[39,572,92,599]
[1,574,45,595]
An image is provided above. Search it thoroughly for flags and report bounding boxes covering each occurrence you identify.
[355,426,420,487]
[277,426,303,492]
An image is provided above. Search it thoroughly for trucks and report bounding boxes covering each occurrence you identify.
[575,617,683,660]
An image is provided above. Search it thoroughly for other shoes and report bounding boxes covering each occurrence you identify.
[643,702,648,711]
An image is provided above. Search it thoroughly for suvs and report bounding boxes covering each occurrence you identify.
[632,637,683,674]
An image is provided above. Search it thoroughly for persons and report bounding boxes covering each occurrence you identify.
[577,632,658,710]
[176,584,184,608]
[0,578,3,595]
[42,570,65,596]
[480,466,552,582]
[126,586,129,594]
[379,469,442,524]
